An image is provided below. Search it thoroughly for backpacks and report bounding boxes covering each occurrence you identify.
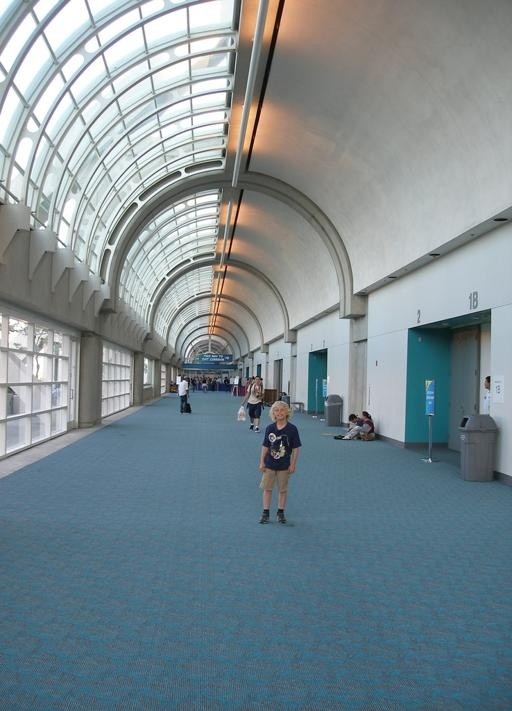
[183,403,191,413]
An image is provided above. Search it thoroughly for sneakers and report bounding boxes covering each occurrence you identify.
[342,435,351,440]
[258,509,287,525]
[249,423,261,433]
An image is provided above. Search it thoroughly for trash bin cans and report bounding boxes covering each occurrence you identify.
[324,395,343,426]
[282,395,290,409]
[458,414,497,483]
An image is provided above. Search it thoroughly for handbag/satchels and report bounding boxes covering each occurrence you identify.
[237,406,247,422]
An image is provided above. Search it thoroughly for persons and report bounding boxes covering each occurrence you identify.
[257,401,302,525]
[241,376,265,434]
[341,412,376,442]
[181,376,230,393]
[348,415,364,441]
[482,376,490,416]
[177,375,190,415]
[236,377,256,397]
